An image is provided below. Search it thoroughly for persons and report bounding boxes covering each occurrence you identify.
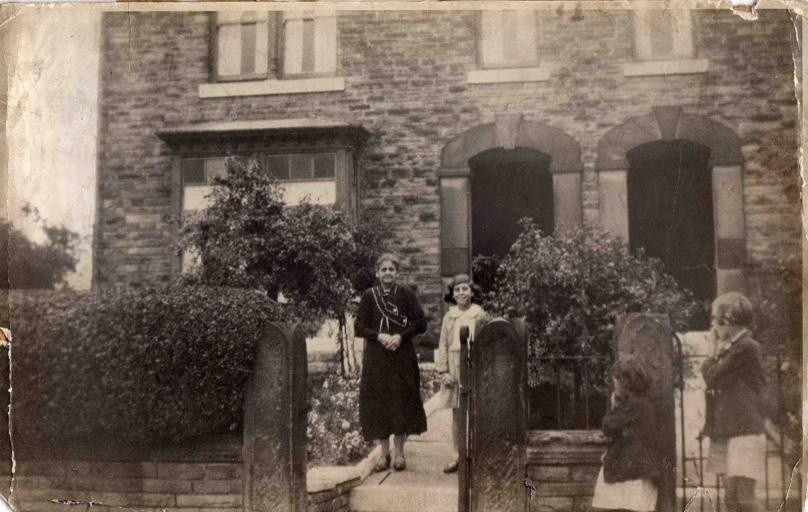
[435,273,491,473]
[355,252,427,471]
[591,356,658,512]
[702,291,763,512]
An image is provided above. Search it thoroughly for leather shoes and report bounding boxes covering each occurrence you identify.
[374,453,405,472]
[444,459,459,472]
